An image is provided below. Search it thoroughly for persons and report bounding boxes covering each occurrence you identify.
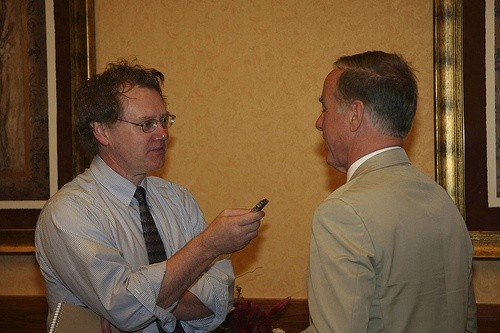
[300,50,476,333]
[34,57,265,333]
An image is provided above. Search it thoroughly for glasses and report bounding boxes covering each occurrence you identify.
[97,113,177,133]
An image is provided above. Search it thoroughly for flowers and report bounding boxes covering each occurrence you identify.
[218,283,293,333]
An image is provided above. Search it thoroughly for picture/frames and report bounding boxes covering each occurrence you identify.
[0,0,97,254]
[431,0,500,259]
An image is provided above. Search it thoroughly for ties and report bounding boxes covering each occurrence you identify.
[131,186,168,265]
